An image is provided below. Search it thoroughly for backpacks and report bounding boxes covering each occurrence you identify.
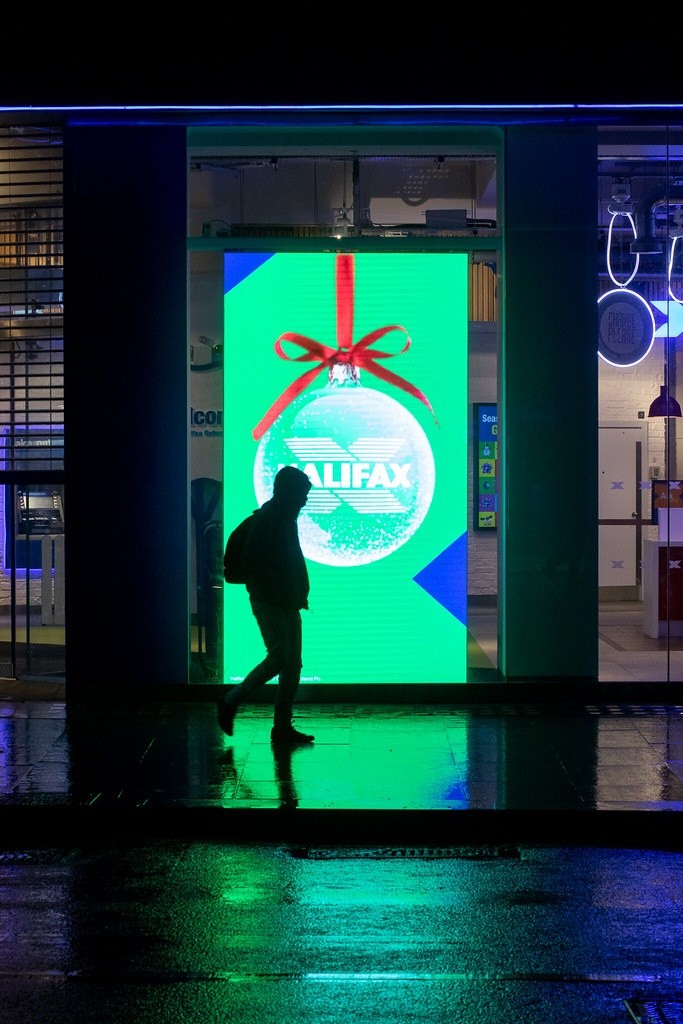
[223,510,262,584]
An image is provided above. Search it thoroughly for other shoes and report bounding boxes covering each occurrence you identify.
[271,720,314,745]
[217,695,236,736]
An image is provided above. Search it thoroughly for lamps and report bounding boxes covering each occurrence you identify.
[648,386,683,418]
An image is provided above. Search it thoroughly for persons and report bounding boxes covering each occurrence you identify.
[219,466,315,745]
